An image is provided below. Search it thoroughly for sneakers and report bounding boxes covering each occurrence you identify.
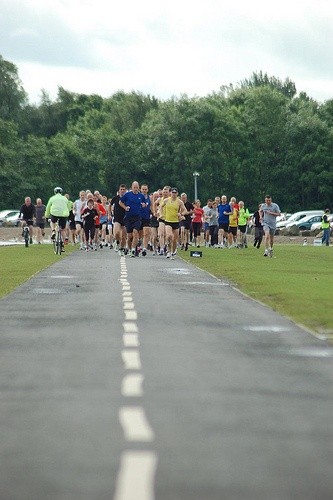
[73,240,274,264]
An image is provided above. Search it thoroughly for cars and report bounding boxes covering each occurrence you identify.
[311,215,333,238]
[0,209,23,227]
[275,213,291,224]
[286,214,323,230]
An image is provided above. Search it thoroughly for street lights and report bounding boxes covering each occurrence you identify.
[193,170,199,203]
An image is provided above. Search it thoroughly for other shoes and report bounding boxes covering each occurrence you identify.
[30,238,33,244]
[51,232,56,239]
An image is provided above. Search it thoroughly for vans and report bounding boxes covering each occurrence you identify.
[275,209,325,232]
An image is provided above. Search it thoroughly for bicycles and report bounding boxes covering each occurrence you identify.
[46,217,68,255]
[17,218,33,247]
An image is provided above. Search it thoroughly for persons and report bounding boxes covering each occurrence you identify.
[45,188,71,248]
[177,193,221,251]
[67,191,88,250]
[139,184,171,256]
[18,197,37,244]
[87,185,130,255]
[224,198,250,248]
[34,198,47,243]
[80,199,99,252]
[321,209,333,247]
[252,204,264,248]
[118,181,147,257]
[259,195,281,257]
[215,195,233,249]
[157,188,187,260]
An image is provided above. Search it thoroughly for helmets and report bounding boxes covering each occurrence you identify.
[54,187,63,194]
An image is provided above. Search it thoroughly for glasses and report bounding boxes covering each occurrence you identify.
[172,192,177,193]
